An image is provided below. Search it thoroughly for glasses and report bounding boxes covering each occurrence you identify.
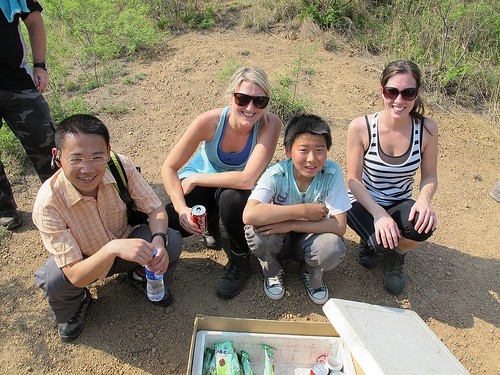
[233,92,270,109]
[382,86,419,102]
[59,153,107,167]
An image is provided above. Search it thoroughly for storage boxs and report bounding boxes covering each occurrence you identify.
[186,298,473,375]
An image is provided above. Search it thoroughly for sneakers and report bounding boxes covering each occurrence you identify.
[357,237,380,268]
[264,266,285,300]
[57,286,92,342]
[129,270,173,307]
[301,268,329,305]
[383,248,406,295]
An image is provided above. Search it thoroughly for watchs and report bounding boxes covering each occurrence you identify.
[152,232,169,249]
[33,63,48,70]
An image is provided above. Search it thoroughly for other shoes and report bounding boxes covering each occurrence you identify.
[0,206,21,229]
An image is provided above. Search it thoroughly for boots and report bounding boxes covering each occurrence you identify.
[216,251,254,299]
[202,220,221,248]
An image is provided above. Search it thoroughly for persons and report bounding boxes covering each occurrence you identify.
[244,114,351,304]
[162,67,281,299]
[33,114,185,341]
[346,60,440,296]
[0,0,62,229]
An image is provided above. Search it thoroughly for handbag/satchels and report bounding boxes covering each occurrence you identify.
[108,150,150,227]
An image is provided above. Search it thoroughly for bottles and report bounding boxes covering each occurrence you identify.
[145,249,164,302]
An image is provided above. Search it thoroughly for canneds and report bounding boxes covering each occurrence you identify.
[190,205,207,234]
[310,356,344,375]
